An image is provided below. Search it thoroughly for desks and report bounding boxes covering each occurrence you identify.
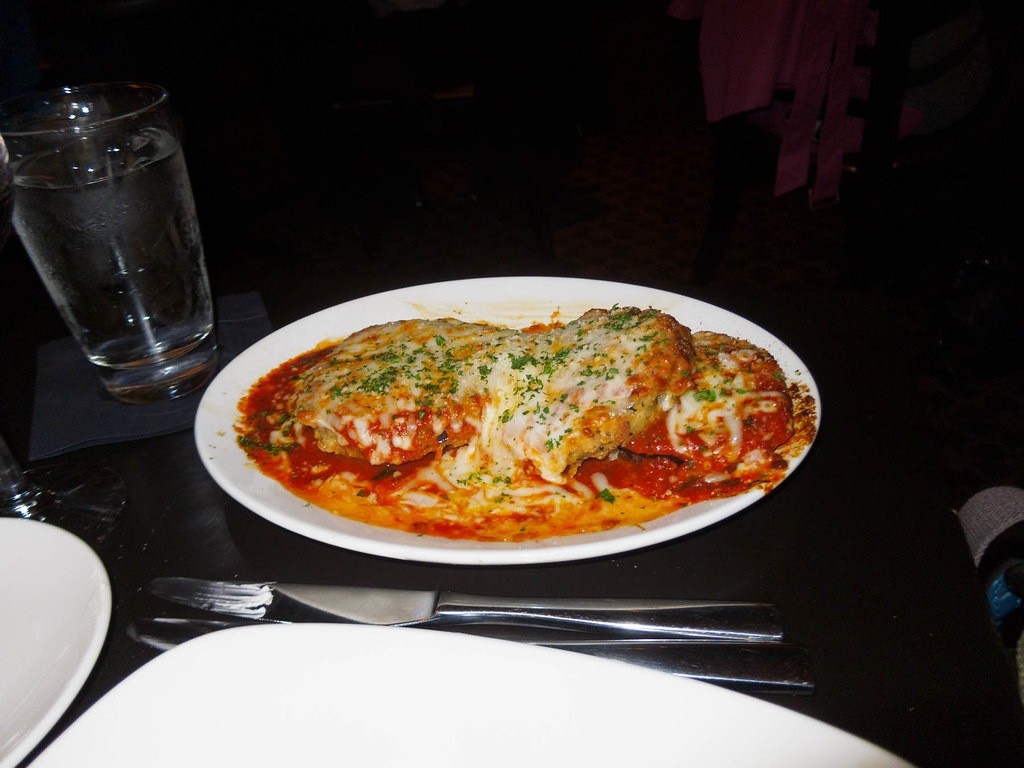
[0,277,1024,768]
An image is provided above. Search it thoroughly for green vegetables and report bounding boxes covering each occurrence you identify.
[238,304,785,502]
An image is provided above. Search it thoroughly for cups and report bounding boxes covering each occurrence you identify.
[0,81,218,404]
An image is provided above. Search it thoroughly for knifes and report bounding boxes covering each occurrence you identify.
[128,577,813,687]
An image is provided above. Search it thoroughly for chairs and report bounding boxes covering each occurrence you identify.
[690,0,994,310]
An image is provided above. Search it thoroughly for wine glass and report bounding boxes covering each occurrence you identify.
[0,134,128,550]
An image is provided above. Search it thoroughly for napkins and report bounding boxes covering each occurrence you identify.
[29,290,274,460]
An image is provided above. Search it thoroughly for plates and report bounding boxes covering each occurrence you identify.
[193,276,821,565]
[26,623,913,768]
[0,517,112,768]
